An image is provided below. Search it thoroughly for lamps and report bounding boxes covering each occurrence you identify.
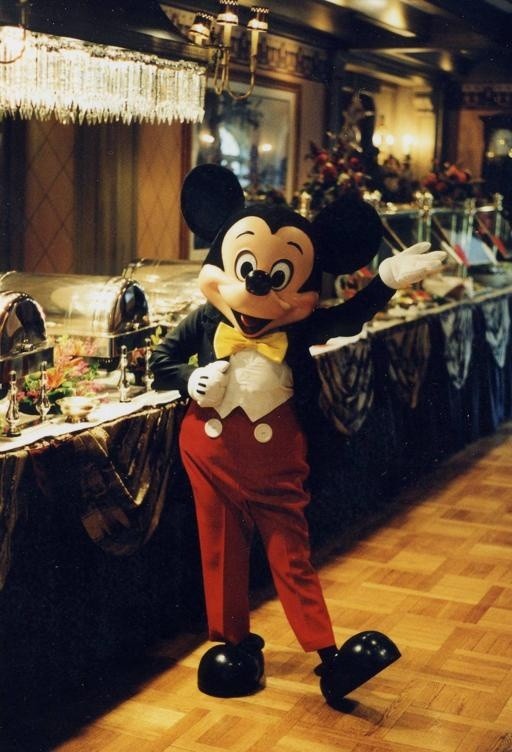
[185,1,273,104]
[1,1,213,130]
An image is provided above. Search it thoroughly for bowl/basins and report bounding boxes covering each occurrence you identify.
[55,397,100,423]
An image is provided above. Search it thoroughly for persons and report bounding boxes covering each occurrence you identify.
[260,151,510,282]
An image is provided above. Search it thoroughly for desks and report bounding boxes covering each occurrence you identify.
[0,276,511,752]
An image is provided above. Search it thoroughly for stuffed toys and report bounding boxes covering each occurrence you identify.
[147,160,448,706]
